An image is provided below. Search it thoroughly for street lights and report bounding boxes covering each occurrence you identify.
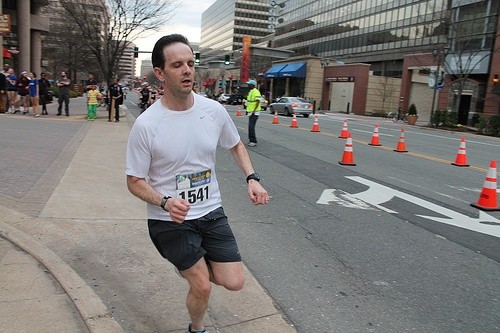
[427,46,450,127]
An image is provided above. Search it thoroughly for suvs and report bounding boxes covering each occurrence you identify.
[217,93,244,105]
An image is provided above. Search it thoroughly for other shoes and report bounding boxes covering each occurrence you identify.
[248,142,257,146]
[23,111,26,115]
[56,113,61,116]
[66,114,69,116]
[188,323,207,333]
[13,111,16,114]
[41,112,45,115]
[16,110,21,112]
[35,114,41,116]
[45,112,48,115]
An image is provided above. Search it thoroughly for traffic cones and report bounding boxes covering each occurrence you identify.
[469,160,500,212]
[338,133,357,166]
[451,137,470,167]
[310,115,321,132]
[236,106,242,116]
[393,128,408,152]
[271,110,279,124]
[289,112,299,128]
[368,124,382,146]
[337,119,349,139]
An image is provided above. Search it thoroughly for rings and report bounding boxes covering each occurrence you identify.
[266,199,269,201]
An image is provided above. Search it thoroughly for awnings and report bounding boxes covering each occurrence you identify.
[264,62,307,78]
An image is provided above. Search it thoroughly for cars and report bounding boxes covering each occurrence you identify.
[242,96,313,118]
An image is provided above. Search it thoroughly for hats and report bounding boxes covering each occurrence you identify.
[21,71,27,74]
[8,68,13,73]
[245,80,256,85]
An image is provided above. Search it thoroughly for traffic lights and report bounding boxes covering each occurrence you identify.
[224,54,230,65]
[195,53,201,63]
[492,74,499,85]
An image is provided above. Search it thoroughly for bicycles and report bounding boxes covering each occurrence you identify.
[392,110,409,123]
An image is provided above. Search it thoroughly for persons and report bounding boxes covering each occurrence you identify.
[244,79,262,147]
[55,71,71,116]
[106,78,123,123]
[85,73,102,121]
[0,63,50,118]
[138,84,157,112]
[125,34,270,333]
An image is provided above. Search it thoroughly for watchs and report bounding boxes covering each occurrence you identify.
[246,172,261,184]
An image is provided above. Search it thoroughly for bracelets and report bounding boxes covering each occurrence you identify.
[160,195,172,212]
